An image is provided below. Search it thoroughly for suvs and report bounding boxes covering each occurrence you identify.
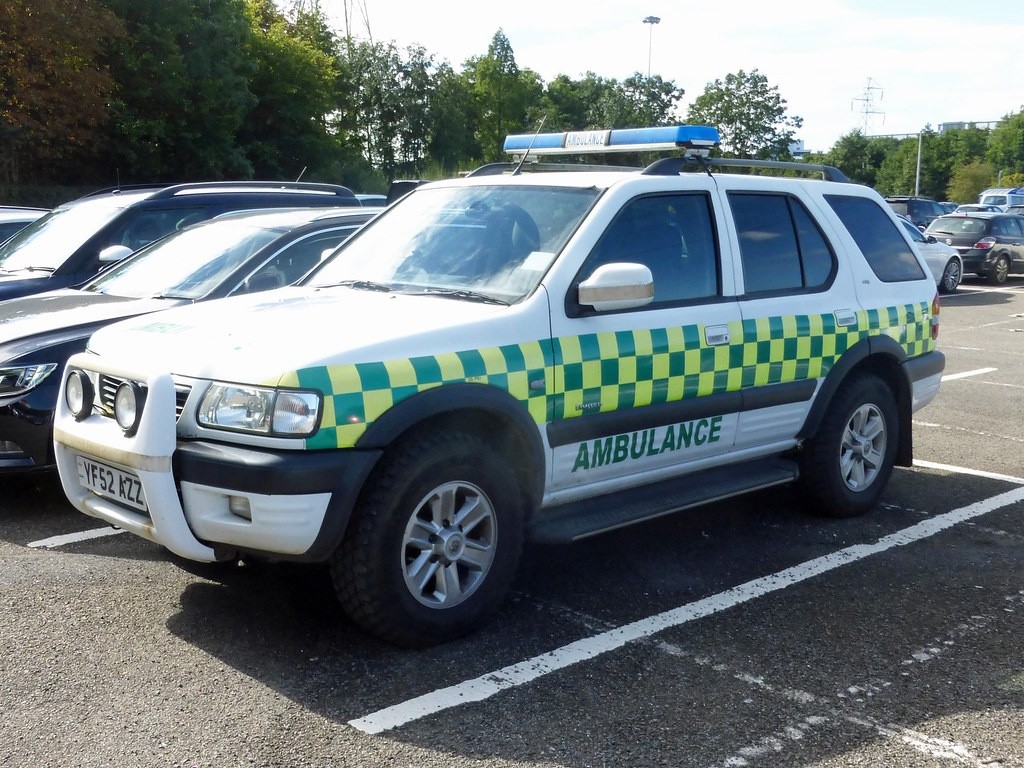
[0,178,359,304]
[54,124,944,648]
[0,206,489,508]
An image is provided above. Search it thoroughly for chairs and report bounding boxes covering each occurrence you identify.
[451,204,541,280]
[600,216,683,300]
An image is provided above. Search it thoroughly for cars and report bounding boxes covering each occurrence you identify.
[883,188,1024,238]
[922,211,1024,286]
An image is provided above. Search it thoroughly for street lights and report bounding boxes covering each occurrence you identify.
[642,16,662,78]
